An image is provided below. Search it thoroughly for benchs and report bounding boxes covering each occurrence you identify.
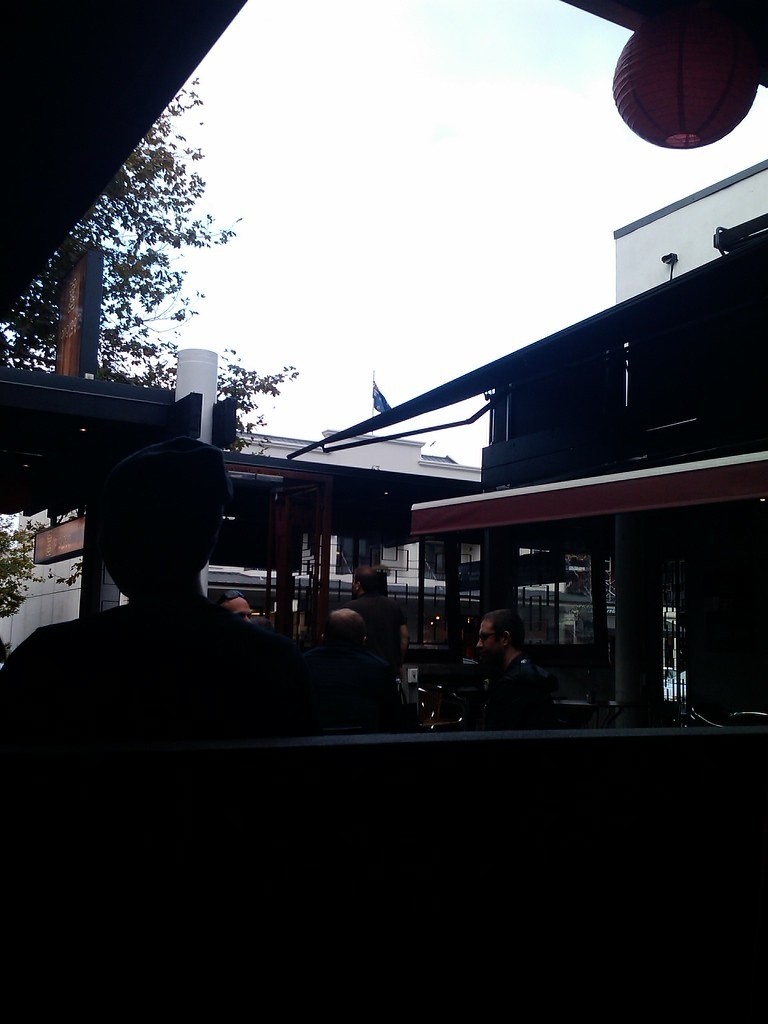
[0,722,768,1024]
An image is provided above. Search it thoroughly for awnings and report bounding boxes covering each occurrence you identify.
[287,236,768,459]
[410,447,767,539]
[4,376,173,523]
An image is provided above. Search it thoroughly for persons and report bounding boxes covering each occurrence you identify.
[464,610,559,731]
[0,436,321,747]
[216,590,252,625]
[340,567,410,668]
[301,608,390,737]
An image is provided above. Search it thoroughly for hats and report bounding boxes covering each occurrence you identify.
[103,437,233,529]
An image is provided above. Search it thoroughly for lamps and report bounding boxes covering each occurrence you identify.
[662,253,678,281]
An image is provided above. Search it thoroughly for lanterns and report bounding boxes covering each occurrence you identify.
[614,7,758,148]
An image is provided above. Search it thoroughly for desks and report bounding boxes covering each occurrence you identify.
[553,699,644,728]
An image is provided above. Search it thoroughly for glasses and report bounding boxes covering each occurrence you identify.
[479,632,495,641]
